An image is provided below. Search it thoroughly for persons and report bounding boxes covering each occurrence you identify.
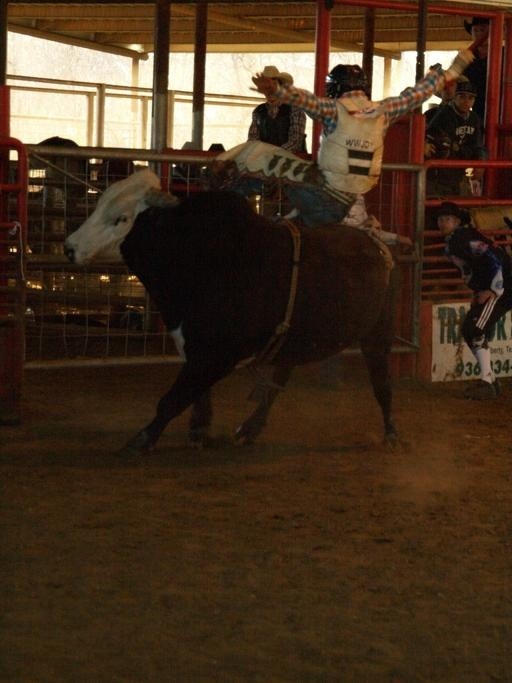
[423,79,486,231]
[202,47,477,230]
[423,80,457,126]
[460,14,491,122]
[435,196,510,400]
[246,64,309,155]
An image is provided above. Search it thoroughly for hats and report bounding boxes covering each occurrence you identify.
[426,199,474,227]
[252,67,293,91]
[453,81,480,98]
[463,14,507,38]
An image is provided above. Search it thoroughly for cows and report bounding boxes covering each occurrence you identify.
[60,164,410,459]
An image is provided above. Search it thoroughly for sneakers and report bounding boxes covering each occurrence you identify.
[454,376,503,400]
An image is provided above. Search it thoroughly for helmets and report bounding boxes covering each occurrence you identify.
[321,63,371,99]
